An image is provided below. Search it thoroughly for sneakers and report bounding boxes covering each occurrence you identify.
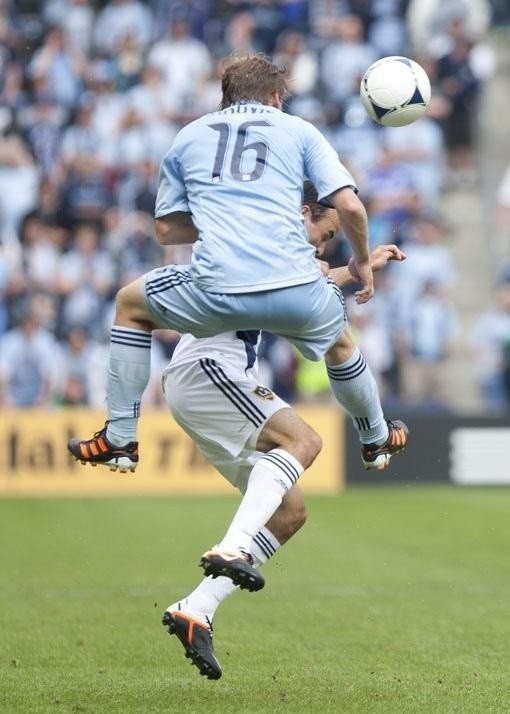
[359,420,410,474]
[67,429,140,476]
[199,545,265,595]
[160,599,223,681]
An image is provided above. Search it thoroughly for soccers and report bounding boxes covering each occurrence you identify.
[359,56,431,128]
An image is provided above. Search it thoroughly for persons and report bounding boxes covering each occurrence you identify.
[160,180,407,679]
[67,55,409,471]
[1,1,510,408]
